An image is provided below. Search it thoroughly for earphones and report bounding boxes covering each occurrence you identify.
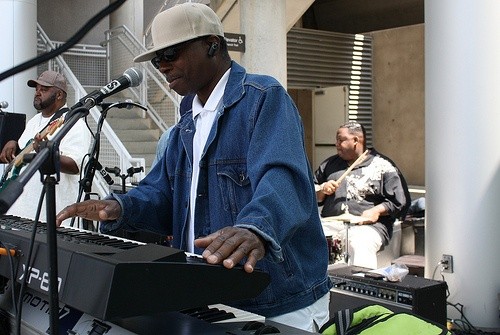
[208,42,217,57]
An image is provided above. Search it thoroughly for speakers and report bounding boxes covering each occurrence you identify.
[328,265,448,330]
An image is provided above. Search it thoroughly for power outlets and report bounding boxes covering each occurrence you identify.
[442,255,452,273]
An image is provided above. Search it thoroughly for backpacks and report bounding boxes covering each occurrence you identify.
[319,304,452,335]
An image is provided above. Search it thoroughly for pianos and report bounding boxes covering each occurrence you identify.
[0,212,324,335]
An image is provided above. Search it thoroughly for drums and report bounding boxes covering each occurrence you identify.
[325,234,342,265]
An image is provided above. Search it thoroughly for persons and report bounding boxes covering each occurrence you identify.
[56,3,334,333]
[0,71,92,229]
[313,123,412,277]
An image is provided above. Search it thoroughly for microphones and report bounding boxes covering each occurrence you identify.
[97,99,134,110]
[88,155,114,186]
[0,101,9,108]
[85,67,144,106]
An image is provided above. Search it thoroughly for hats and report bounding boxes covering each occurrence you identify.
[27,70,68,93]
[132,3,224,62]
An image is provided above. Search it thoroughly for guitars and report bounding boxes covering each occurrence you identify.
[0,115,64,192]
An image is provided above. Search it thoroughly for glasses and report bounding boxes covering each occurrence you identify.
[150,38,209,69]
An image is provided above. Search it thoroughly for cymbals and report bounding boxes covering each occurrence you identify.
[320,213,372,222]
